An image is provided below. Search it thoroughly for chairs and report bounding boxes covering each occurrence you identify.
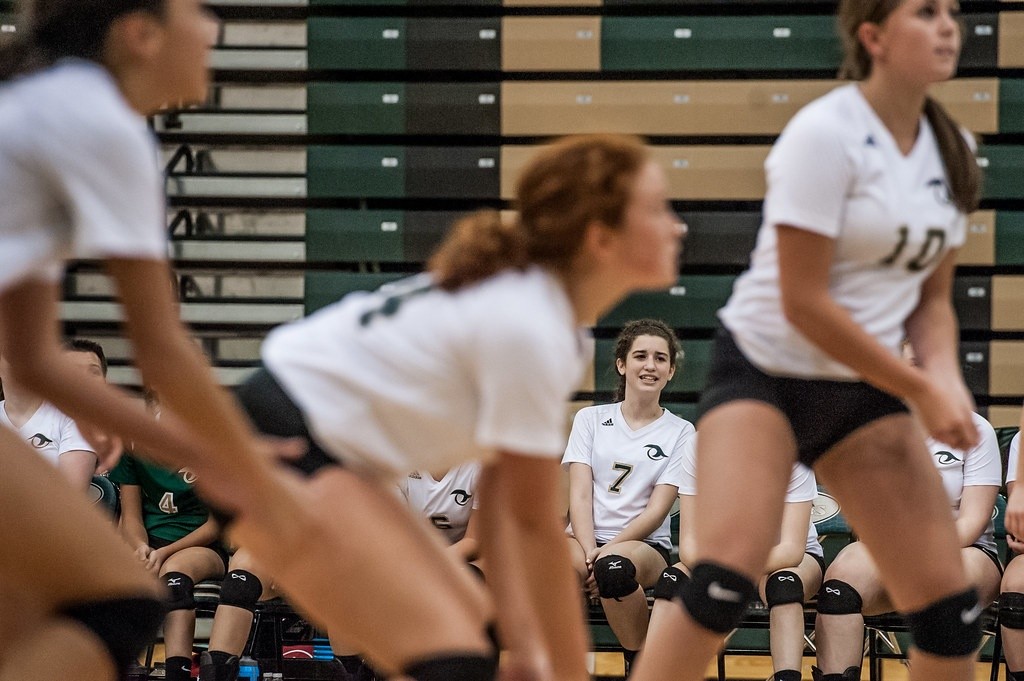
[65,426,1019,681]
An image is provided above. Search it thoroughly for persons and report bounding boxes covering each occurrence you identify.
[625,2,1024,681]
[0,0,375,681]
[183,131,695,681]
